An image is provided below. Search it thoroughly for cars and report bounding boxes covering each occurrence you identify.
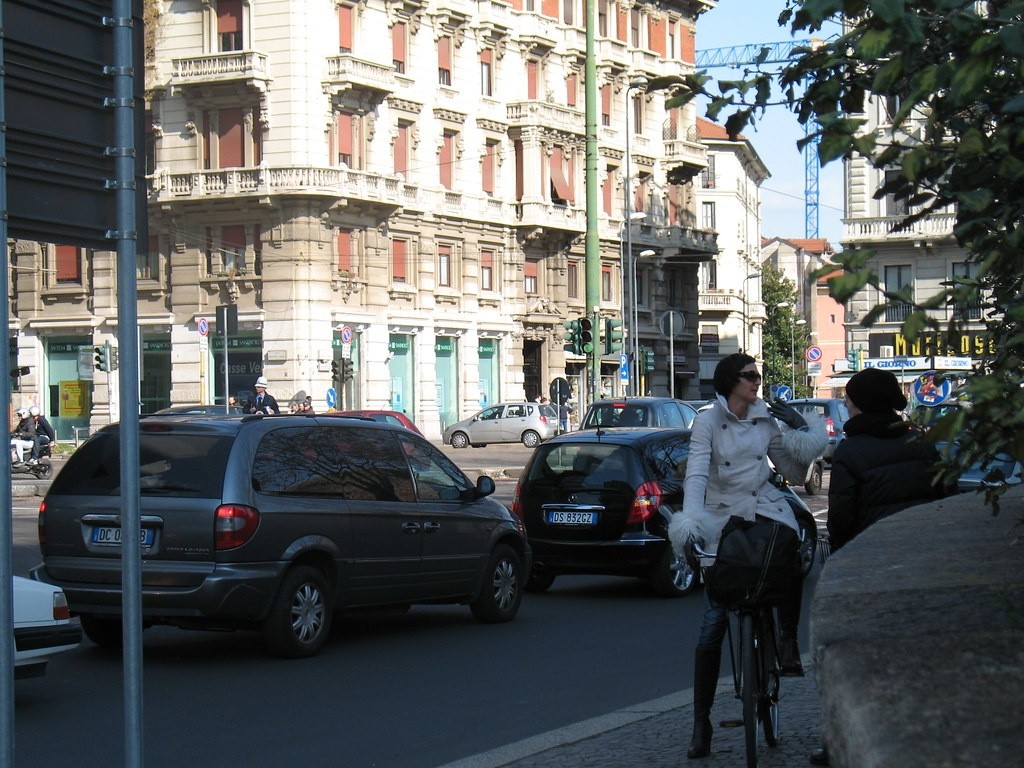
[511,427,819,599]
[578,396,697,456]
[935,440,1023,489]
[784,398,849,461]
[14,574,83,681]
[688,399,824,496]
[300,410,431,479]
[155,404,245,415]
[442,401,561,450]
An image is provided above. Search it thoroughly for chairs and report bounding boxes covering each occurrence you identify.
[509,410,514,415]
[515,410,522,416]
[619,410,640,425]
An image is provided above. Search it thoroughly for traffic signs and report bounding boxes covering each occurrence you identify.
[619,354,629,386]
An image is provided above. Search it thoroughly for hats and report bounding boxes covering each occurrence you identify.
[845,368,907,414]
[255,377,268,387]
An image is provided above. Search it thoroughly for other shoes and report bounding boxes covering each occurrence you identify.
[26,458,38,465]
[12,461,26,468]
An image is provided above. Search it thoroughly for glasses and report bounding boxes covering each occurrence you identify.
[737,371,761,382]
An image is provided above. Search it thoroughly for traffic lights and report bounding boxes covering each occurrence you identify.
[562,320,582,356]
[331,359,343,382]
[847,349,858,371]
[343,358,354,382]
[605,318,622,355]
[94,345,108,372]
[580,317,594,354]
[641,349,655,376]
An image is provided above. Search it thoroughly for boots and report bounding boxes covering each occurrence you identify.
[688,647,721,757]
[775,563,802,671]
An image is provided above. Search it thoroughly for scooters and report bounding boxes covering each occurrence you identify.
[9,433,53,480]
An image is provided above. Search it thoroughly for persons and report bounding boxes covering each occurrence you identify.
[535,395,573,432]
[667,353,828,758]
[230,396,240,406]
[11,407,55,467]
[826,368,958,558]
[288,396,315,414]
[243,377,281,414]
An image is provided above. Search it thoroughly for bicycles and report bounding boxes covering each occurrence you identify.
[687,535,805,768]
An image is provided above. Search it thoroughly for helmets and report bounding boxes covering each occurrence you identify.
[17,409,29,419]
[29,407,39,416]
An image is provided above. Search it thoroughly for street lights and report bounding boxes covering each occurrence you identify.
[633,248,658,398]
[791,319,806,400]
[625,77,649,397]
[805,331,818,398]
[619,211,648,397]
[772,302,789,385]
[742,273,762,355]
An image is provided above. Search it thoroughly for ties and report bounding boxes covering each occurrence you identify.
[257,397,262,409]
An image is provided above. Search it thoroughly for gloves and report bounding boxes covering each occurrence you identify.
[768,397,808,430]
[684,534,705,570]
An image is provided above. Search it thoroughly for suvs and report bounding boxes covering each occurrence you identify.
[28,411,532,663]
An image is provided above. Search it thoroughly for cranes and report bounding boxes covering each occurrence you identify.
[694,38,829,240]
